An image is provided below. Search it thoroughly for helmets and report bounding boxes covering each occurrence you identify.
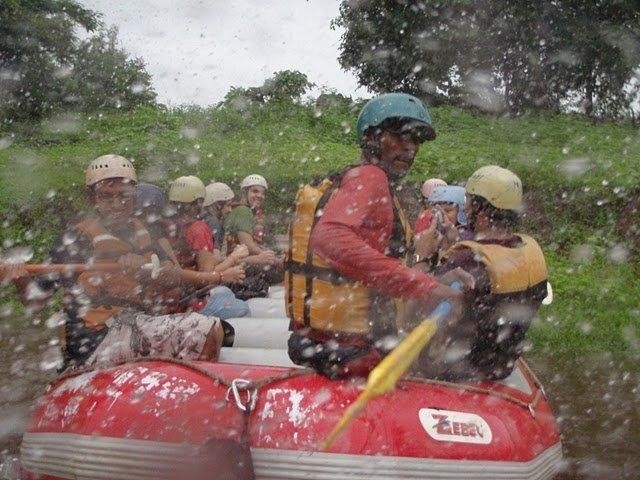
[135,184,168,214]
[240,174,268,190]
[85,154,137,188]
[168,176,206,203]
[465,165,524,213]
[202,182,234,208]
[357,92,436,144]
[428,185,469,226]
[421,178,446,199]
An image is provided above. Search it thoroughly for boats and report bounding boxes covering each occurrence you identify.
[20,280,565,474]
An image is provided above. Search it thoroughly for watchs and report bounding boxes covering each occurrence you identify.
[407,253,430,267]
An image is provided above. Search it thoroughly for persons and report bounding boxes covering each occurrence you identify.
[410,163,548,384]
[417,177,445,234]
[0,153,225,363]
[286,92,475,379]
[132,173,285,319]
[419,184,473,256]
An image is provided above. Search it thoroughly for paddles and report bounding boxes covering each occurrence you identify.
[320,282,461,451]
